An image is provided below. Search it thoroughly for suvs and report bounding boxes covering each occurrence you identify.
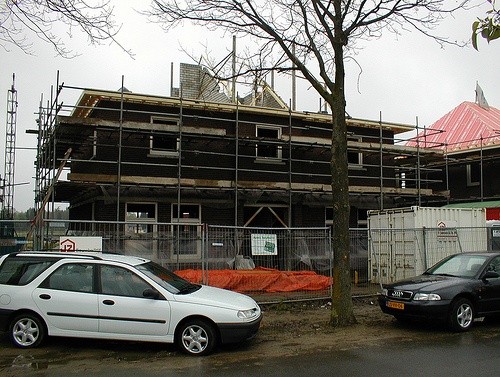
[0,250,264,356]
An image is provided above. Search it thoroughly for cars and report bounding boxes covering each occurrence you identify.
[376,251,500,332]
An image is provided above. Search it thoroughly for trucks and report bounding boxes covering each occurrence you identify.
[367,206,500,288]
